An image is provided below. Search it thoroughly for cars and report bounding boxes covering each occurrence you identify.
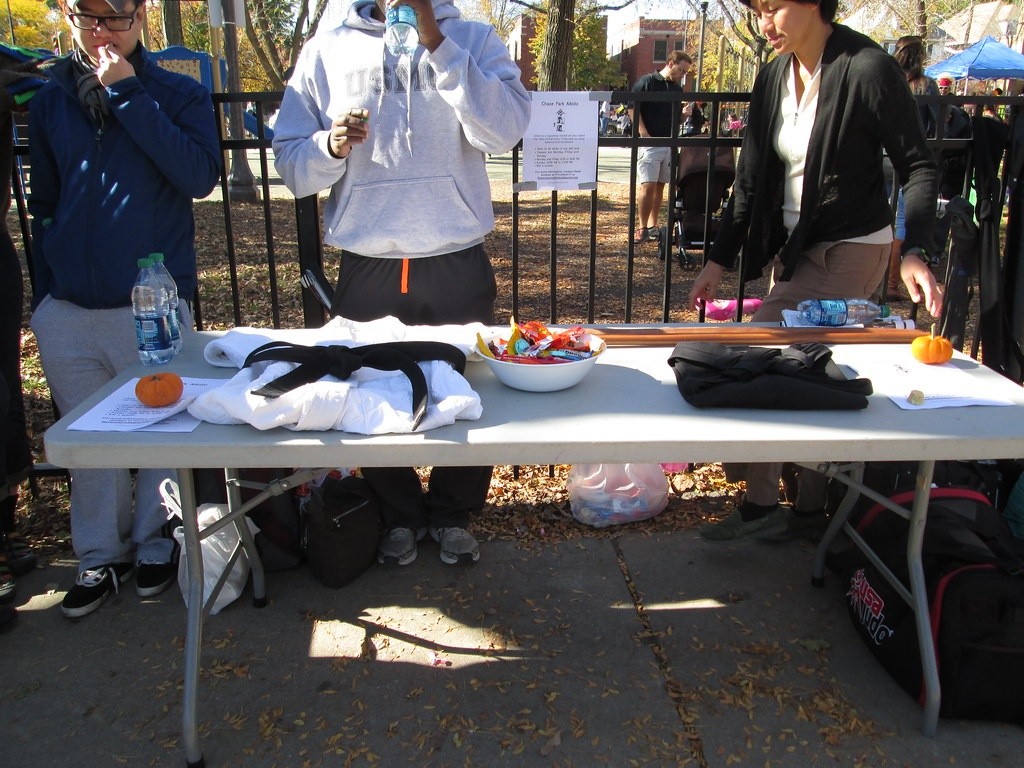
[607,104,628,135]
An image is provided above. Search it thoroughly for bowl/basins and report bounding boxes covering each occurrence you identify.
[474,328,608,392]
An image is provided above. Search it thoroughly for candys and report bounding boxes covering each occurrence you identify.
[486,321,601,364]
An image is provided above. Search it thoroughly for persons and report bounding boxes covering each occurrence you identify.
[594,37,1024,243]
[271,0,530,567]
[29,0,222,619]
[686,0,942,543]
[0,87,36,597]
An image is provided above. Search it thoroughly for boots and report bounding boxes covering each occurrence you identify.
[886,239,909,300]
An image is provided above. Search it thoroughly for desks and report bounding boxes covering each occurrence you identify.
[45,326,1024,768]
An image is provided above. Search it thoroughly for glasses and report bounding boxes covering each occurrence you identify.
[678,68,688,76]
[939,87,948,89]
[62,1,141,32]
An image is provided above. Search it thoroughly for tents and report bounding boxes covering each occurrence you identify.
[923,34,1023,97]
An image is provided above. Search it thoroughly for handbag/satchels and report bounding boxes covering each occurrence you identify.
[298,476,383,590]
[568,462,670,529]
[599,111,603,118]
[837,483,1024,725]
[160,477,262,615]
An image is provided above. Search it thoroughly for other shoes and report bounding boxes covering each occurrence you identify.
[0,549,17,601]
[634,227,649,244]
[648,226,660,241]
[8,528,36,570]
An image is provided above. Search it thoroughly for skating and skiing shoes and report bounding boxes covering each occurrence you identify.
[252,468,302,570]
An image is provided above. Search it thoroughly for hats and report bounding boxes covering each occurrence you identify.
[66,0,129,14]
[938,78,952,86]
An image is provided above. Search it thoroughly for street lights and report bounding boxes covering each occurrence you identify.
[695,1,708,93]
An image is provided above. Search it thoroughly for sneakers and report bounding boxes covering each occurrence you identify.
[760,508,829,543]
[135,560,174,597]
[377,525,429,566]
[428,525,480,564]
[698,505,790,544]
[61,562,134,617]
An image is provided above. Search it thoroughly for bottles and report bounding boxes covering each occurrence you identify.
[384,0,418,57]
[131,257,174,366]
[148,253,182,355]
[796,298,890,327]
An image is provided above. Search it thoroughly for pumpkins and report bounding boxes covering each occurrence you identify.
[135,372,183,407]
[911,323,953,363]
